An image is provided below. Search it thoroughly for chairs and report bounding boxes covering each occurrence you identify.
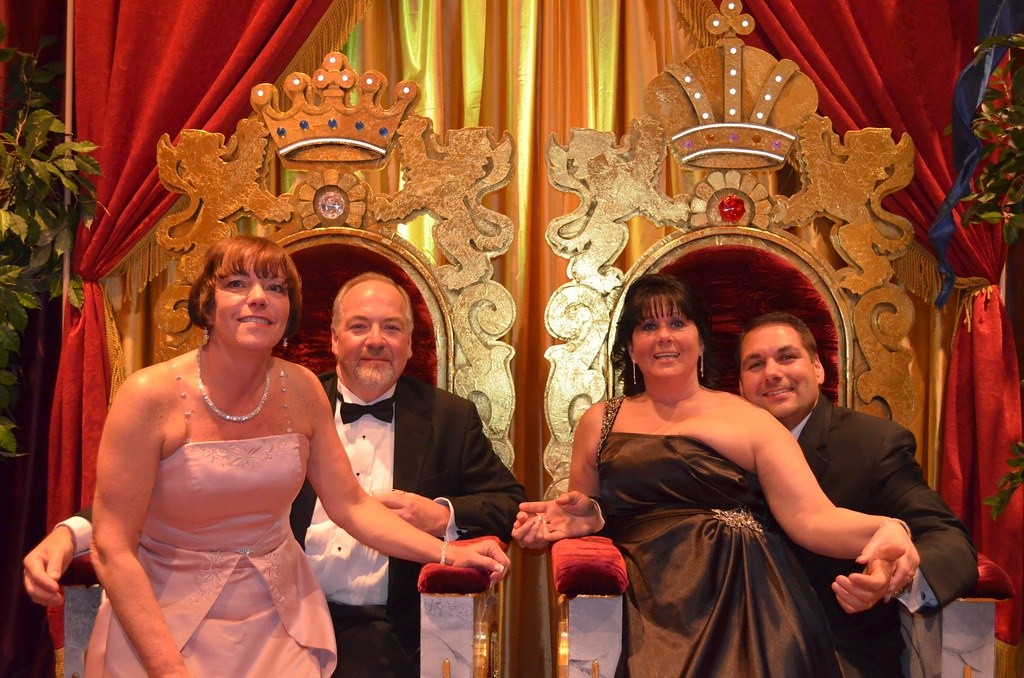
[28,52,521,678]
[539,0,1016,678]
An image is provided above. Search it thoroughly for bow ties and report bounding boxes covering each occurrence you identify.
[340,402,393,425]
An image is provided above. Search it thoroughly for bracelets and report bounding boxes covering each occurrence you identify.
[892,576,916,598]
[880,517,912,538]
[441,542,448,564]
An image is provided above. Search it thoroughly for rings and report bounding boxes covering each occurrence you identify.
[518,541,525,548]
[867,600,873,609]
[538,515,543,523]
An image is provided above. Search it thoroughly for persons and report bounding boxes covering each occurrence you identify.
[89,237,511,678]
[24,269,527,678]
[512,276,922,678]
[739,314,978,678]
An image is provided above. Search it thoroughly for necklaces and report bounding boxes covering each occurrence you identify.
[197,344,270,422]
